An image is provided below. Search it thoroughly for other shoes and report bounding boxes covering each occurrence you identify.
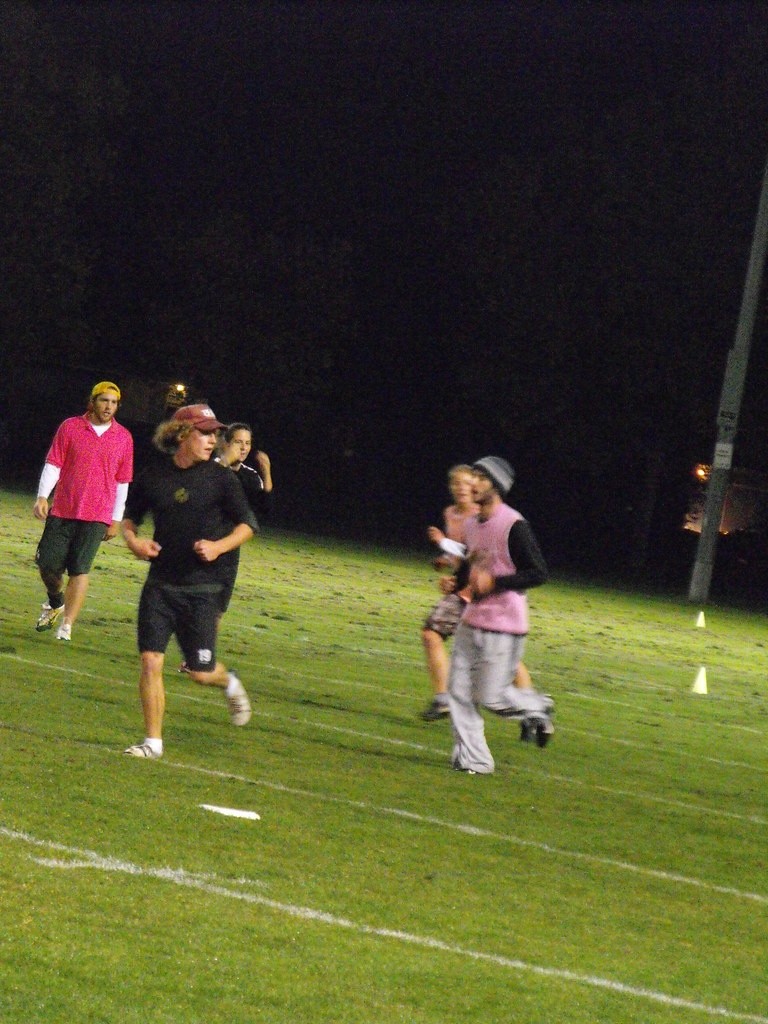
[452,757,477,774]
[420,700,450,722]
[178,662,191,674]
[534,695,555,748]
[520,719,536,742]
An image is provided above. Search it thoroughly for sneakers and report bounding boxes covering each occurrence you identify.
[123,744,166,759]
[224,676,252,726]
[36,603,66,632]
[56,617,72,641]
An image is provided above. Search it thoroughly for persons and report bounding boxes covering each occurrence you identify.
[177,423,274,675]
[34,381,134,642]
[419,447,556,776]
[121,403,261,759]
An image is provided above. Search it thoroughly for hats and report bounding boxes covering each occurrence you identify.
[92,381,121,401]
[171,404,228,432]
[474,457,515,495]
